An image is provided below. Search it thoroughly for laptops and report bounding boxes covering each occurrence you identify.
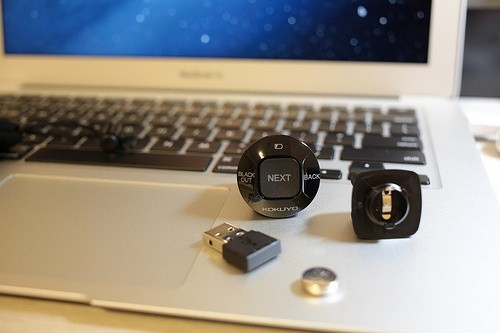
[0,1,499,331]
